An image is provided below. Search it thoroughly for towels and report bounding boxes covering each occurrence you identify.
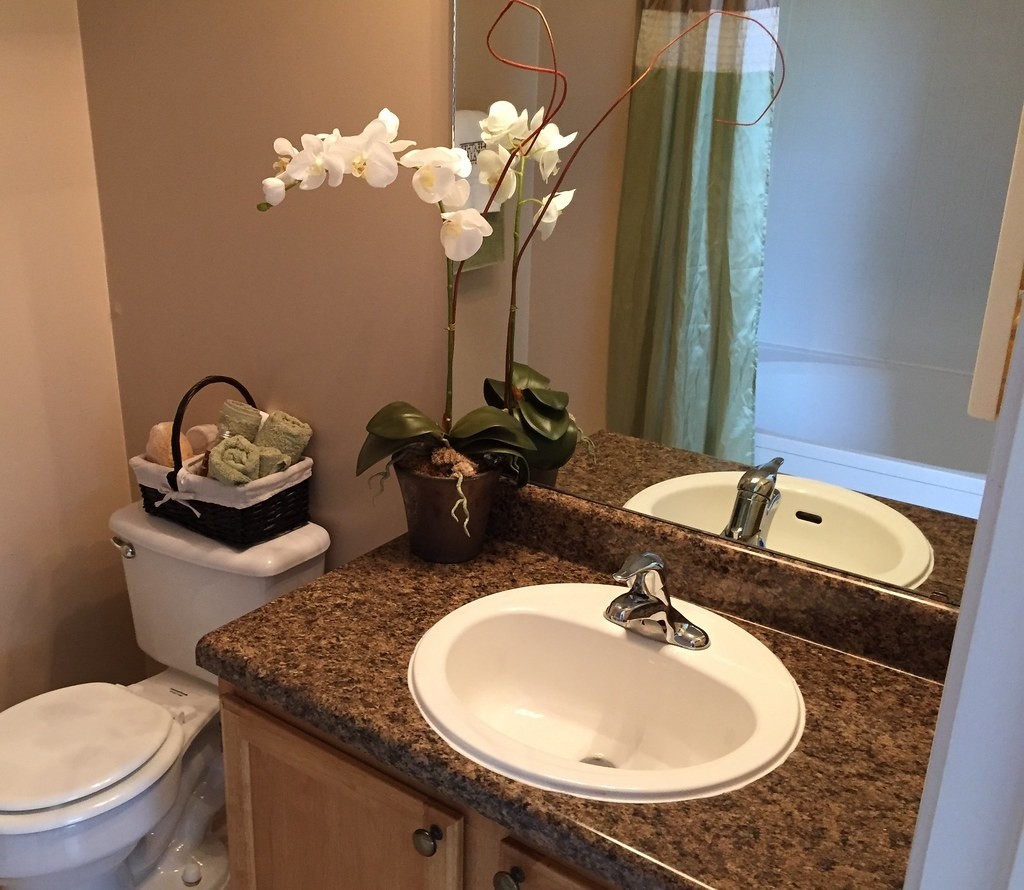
[207,399,314,487]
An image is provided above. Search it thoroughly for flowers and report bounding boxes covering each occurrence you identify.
[254,99,537,489]
[475,101,579,471]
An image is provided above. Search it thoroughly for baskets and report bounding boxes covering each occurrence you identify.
[129,376,313,551]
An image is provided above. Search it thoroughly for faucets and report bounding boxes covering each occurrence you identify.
[720,457,785,548]
[604,550,709,651]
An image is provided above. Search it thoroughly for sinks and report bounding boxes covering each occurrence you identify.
[621,470,935,590]
[407,583,808,804]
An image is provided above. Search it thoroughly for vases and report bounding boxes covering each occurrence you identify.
[537,467,561,488]
[389,451,500,565]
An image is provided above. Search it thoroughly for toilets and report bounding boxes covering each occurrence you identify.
[0,495,332,890]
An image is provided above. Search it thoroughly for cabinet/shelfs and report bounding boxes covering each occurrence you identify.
[215,678,616,889]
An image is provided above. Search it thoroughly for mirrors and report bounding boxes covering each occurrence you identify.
[450,0,1024,604]
